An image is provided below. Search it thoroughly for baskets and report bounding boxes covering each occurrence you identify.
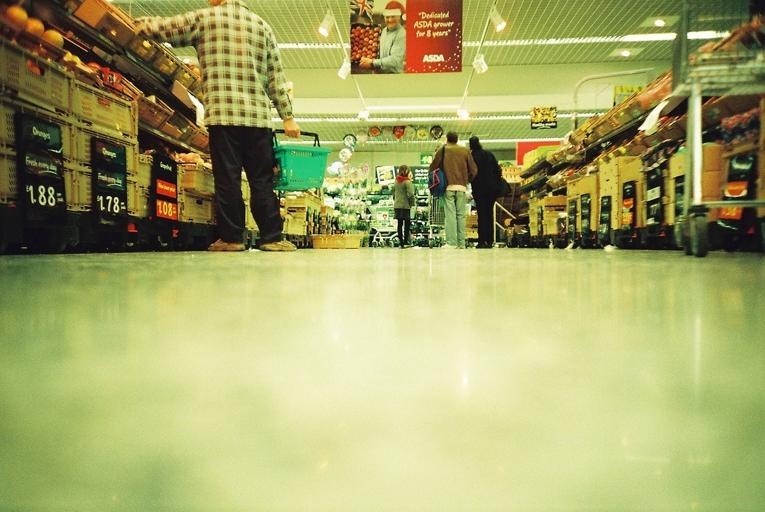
[311,231,364,250]
[272,128,332,190]
[1,0,218,226]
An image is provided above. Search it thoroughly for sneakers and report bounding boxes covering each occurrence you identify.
[260,240,296,251]
[208,239,245,251]
[442,244,465,249]
[476,242,493,248]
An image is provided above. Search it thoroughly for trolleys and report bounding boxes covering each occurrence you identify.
[362,193,446,249]
[666,2,765,255]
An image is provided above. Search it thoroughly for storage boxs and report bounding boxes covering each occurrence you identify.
[528,142,724,237]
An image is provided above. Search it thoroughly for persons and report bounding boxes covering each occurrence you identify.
[429,130,479,250]
[391,164,416,250]
[468,135,503,249]
[358,1,405,75]
[133,0,302,253]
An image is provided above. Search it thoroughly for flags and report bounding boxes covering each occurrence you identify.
[349,0,374,24]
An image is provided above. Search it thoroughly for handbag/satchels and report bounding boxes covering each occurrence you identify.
[495,178,511,198]
[427,168,446,198]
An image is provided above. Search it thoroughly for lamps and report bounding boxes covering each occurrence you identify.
[318,9,370,120]
[472,4,507,75]
